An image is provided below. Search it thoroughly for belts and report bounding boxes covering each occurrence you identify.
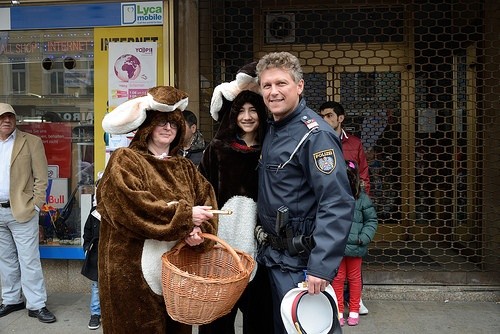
[0,199,11,209]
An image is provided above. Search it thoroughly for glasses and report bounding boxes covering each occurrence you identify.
[157,119,181,128]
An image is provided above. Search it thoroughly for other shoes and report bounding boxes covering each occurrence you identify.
[338,312,345,326]
[359,299,369,314]
[347,311,360,325]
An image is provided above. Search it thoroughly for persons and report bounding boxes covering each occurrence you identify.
[330,160,378,327]
[176,111,205,171]
[254,52,356,334]
[0,103,57,324]
[318,101,371,315]
[96,85,218,334]
[197,61,274,334]
[80,180,103,329]
[68,148,94,184]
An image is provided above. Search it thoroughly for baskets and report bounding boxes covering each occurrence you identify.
[161,233,256,325]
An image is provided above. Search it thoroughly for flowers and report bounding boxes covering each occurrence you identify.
[348,162,357,169]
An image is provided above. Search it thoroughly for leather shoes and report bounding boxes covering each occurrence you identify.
[88,314,101,329]
[0,302,25,316]
[28,306,56,323]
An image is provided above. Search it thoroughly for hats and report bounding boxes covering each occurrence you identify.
[0,103,16,117]
[280,281,344,334]
